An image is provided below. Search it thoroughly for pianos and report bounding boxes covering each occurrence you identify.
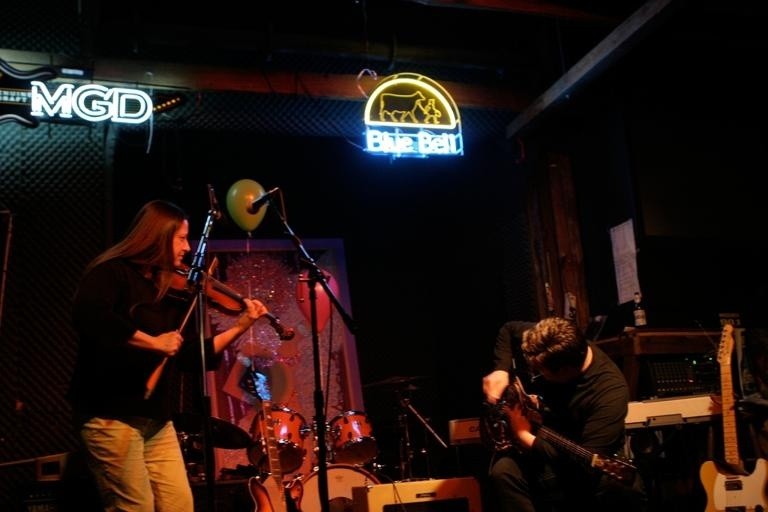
[449,393,720,446]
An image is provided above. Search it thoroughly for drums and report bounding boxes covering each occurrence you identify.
[301,463,380,512]
[328,411,379,466]
[245,408,307,474]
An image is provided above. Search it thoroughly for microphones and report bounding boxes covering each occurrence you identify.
[246,187,281,214]
[186,208,218,289]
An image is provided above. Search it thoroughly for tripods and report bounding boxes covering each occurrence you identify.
[378,401,447,477]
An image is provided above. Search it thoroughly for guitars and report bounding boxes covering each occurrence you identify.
[248,372,303,512]
[698,325,768,512]
[483,375,636,485]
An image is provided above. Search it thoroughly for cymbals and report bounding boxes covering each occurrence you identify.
[210,416,253,449]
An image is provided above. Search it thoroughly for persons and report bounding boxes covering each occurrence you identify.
[482,317,629,512]
[68,200,269,512]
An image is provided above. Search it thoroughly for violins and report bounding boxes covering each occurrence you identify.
[154,262,294,340]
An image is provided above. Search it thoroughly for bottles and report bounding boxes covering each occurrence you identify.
[632,293,647,329]
